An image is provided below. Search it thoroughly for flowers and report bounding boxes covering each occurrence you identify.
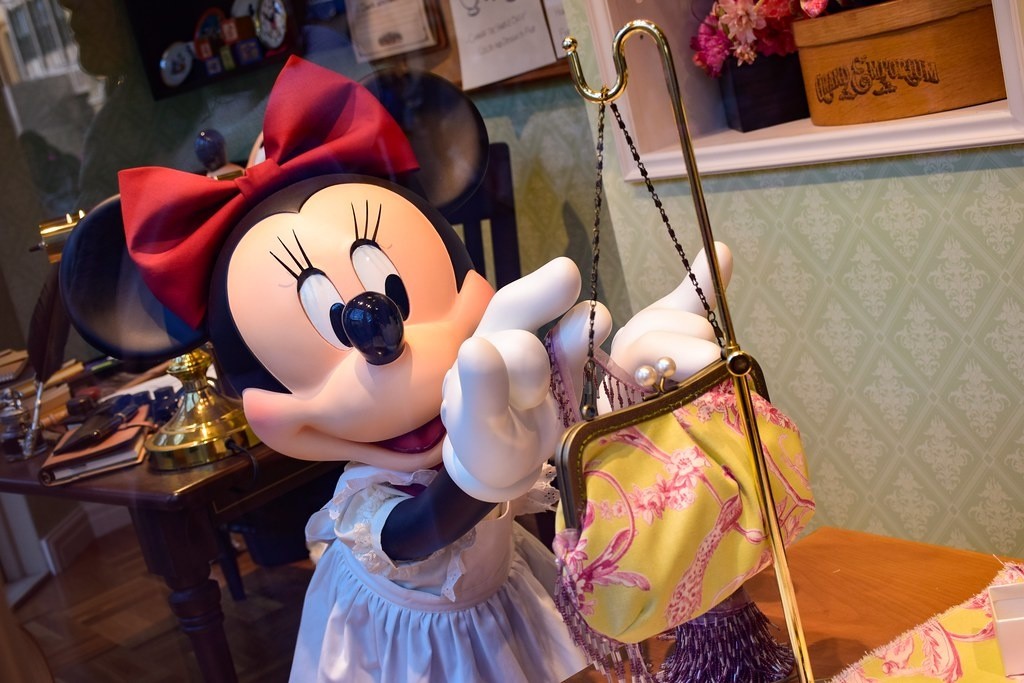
[691,0,842,79]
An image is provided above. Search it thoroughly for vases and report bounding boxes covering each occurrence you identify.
[719,53,809,133]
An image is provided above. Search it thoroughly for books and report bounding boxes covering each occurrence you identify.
[38,403,149,487]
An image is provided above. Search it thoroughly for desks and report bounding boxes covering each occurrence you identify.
[1,418,344,682]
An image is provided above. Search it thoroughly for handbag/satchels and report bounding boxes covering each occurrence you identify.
[551,88,812,649]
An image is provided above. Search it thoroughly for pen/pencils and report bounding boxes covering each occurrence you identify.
[94,404,139,439]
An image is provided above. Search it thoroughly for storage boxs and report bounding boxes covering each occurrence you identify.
[797,0,1009,128]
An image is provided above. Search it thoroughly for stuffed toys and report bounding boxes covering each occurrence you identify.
[55,53,733,683]
